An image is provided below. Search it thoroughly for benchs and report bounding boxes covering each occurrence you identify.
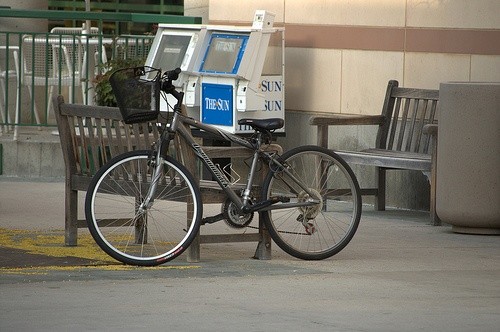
[52,94,283,263]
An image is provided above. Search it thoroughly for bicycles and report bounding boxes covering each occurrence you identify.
[84,66,362,266]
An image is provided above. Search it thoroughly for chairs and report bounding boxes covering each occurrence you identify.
[0,46,41,137]
[109,33,155,71]
[13,33,89,142]
[46,26,108,128]
[309,79,439,226]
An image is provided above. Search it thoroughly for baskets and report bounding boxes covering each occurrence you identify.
[109,66,162,124]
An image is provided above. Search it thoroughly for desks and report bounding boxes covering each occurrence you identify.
[25,38,152,129]
